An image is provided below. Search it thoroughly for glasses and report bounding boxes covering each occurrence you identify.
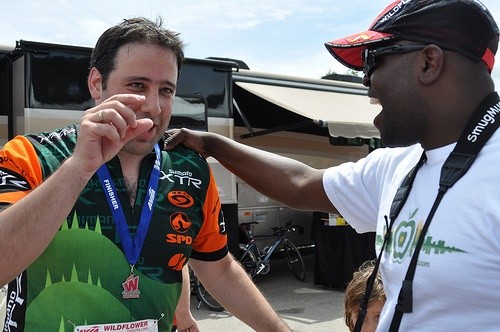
[362,44,426,77]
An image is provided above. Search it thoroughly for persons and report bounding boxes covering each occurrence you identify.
[165,1,496,331]
[344,262,386,331]
[1,17,290,332]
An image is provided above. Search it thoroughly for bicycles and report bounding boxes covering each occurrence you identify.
[197,264,229,311]
[234,221,306,285]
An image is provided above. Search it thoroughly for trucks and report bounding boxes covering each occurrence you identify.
[1,39,380,286]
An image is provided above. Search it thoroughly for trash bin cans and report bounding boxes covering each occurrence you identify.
[312,217,357,289]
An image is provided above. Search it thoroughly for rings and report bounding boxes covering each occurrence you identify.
[98,110,104,122]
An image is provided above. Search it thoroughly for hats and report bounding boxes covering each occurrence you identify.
[324,0,500,71]
